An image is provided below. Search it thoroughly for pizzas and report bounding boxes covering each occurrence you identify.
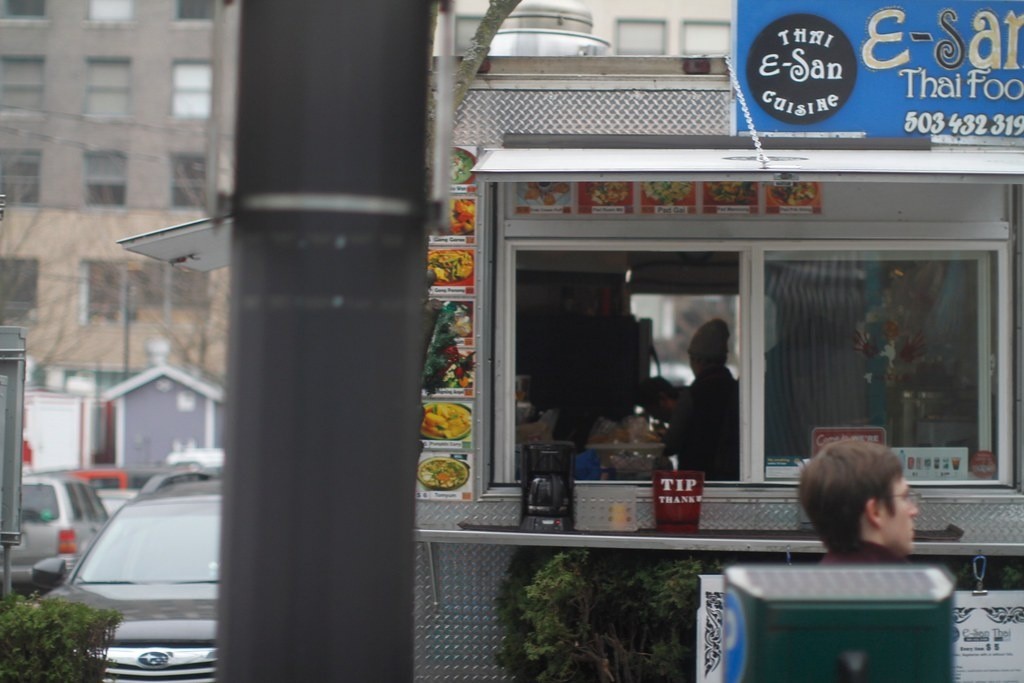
[417,458,468,490]
[768,182,820,207]
[643,182,694,204]
[427,249,473,281]
[449,199,476,234]
[449,148,474,185]
[588,183,630,204]
[707,182,757,204]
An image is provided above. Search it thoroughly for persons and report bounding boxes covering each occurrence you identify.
[645,379,688,423]
[800,441,916,565]
[664,317,740,481]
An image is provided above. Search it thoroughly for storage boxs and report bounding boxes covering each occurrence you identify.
[584,443,666,471]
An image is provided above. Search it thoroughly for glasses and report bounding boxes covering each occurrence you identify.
[889,490,921,507]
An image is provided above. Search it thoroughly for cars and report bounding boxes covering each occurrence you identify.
[78,469,145,516]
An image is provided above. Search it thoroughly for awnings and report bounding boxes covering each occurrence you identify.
[115,217,230,273]
[470,146,1024,184]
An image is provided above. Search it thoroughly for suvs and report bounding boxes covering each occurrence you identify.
[29,472,221,683]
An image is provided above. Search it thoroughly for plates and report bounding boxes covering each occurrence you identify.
[416,148,478,491]
[511,179,820,211]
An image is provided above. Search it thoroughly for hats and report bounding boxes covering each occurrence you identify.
[687,319,730,364]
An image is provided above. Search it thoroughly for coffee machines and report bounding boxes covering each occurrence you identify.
[520,441,577,534]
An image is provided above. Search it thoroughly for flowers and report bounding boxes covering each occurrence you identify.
[852,297,909,385]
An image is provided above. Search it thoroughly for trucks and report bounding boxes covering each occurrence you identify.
[16,388,114,475]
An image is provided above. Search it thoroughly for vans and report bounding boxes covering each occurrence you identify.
[7,474,109,598]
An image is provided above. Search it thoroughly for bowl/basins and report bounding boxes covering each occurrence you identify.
[585,442,665,479]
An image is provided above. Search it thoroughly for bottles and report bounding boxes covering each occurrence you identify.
[899,450,906,469]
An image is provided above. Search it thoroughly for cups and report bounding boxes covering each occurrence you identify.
[651,468,704,534]
[952,457,960,470]
